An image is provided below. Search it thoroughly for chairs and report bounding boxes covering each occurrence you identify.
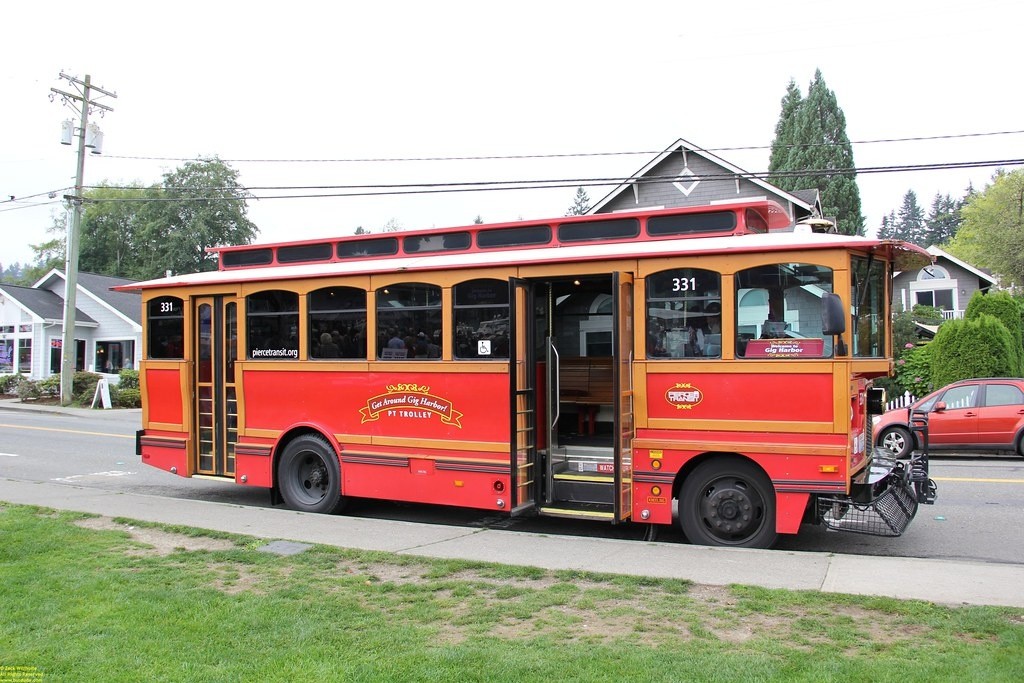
[559,354,590,437]
[576,357,613,440]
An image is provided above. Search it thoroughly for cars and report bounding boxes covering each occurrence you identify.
[871,377,1024,460]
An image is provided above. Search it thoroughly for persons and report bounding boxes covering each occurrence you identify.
[694,302,722,356]
[246,317,510,359]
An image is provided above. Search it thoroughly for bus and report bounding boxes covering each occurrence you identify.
[107,199,938,550]
